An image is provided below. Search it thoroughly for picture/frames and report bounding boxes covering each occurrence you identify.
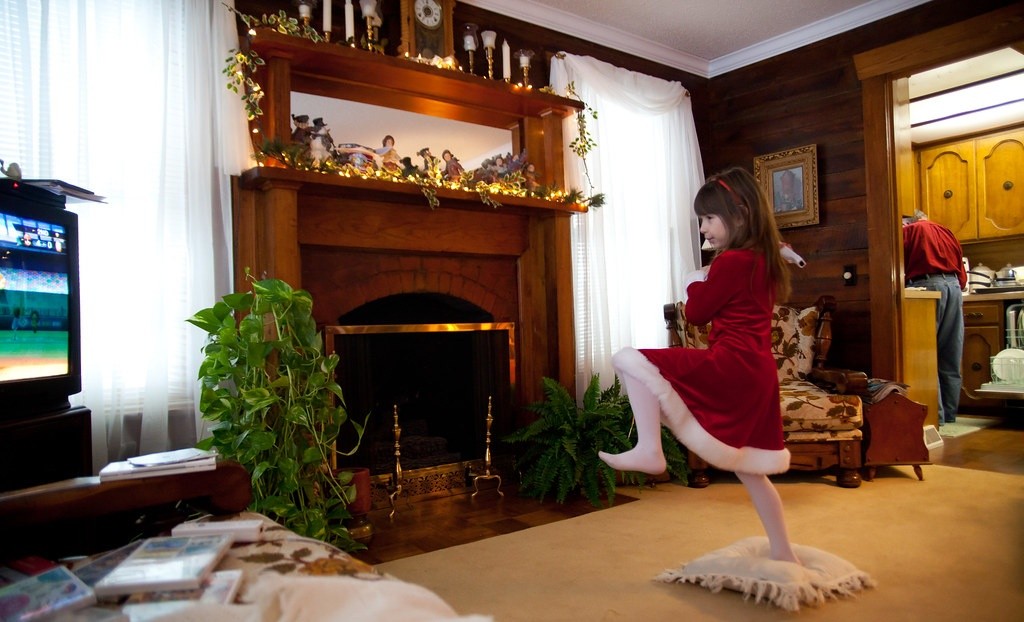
[753,144,820,229]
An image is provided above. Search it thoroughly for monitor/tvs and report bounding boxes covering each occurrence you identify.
[0,192,81,422]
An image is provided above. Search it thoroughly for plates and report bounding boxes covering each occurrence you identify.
[992,348,1024,381]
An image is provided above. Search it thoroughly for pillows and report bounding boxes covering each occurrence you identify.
[653,534,878,614]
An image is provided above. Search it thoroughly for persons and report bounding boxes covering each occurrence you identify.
[598,169,798,562]
[373,135,404,170]
[443,149,464,182]
[902,209,968,426]
[293,115,314,142]
[420,147,442,180]
[490,153,536,191]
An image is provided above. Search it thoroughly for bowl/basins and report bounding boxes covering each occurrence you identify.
[969,262,995,292]
[996,262,1014,279]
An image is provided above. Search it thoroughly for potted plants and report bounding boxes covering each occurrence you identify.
[499,374,691,510]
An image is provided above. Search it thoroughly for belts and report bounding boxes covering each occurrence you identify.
[905,274,956,285]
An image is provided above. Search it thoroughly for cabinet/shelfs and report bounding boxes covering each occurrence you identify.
[917,126,1024,256]
[958,300,1022,417]
[0,405,93,494]
[905,298,939,433]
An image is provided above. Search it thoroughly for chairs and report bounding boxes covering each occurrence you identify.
[664,295,869,489]
[0,458,495,622]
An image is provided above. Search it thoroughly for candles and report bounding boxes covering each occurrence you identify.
[520,56,528,67]
[299,5,310,18]
[463,35,476,50]
[323,0,331,32]
[502,38,511,78]
[345,0,354,47]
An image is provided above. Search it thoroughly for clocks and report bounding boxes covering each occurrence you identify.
[396,0,460,72]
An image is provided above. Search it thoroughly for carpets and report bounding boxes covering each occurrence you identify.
[938,416,993,439]
